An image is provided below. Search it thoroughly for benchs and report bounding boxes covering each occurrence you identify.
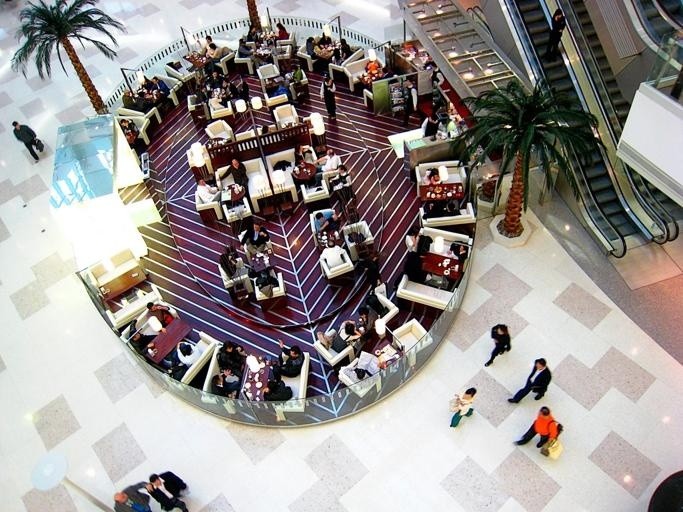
[103,30,236,148]
[203,340,311,416]
[298,29,410,111]
[105,285,220,390]
[392,156,478,314]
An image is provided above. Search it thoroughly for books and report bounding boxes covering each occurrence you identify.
[391,82,404,116]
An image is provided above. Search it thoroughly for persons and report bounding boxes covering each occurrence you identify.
[119,118,141,137]
[320,76,337,120]
[430,63,444,87]
[197,72,248,108]
[239,221,269,254]
[421,114,446,137]
[129,302,174,356]
[424,167,440,186]
[404,225,421,267]
[341,365,379,390]
[544,8,566,63]
[197,179,221,204]
[219,253,247,279]
[331,306,379,353]
[422,200,461,220]
[11,120,44,163]
[366,58,393,79]
[262,338,304,407]
[449,388,477,427]
[315,210,338,232]
[210,340,245,401]
[121,75,170,104]
[238,22,287,58]
[514,406,559,448]
[273,64,302,99]
[216,159,256,215]
[319,240,346,268]
[112,471,189,512]
[401,78,428,127]
[255,270,278,289]
[508,358,552,404]
[485,324,511,366]
[294,145,356,202]
[199,35,223,62]
[306,31,352,66]
[446,241,469,263]
[175,340,202,367]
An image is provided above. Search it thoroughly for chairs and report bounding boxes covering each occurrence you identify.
[188,65,352,230]
[219,225,287,313]
[312,292,433,399]
[310,209,374,282]
[228,24,297,77]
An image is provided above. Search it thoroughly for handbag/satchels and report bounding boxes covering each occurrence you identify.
[540,439,563,461]
[35,139,44,150]
[449,398,458,412]
[548,420,563,439]
